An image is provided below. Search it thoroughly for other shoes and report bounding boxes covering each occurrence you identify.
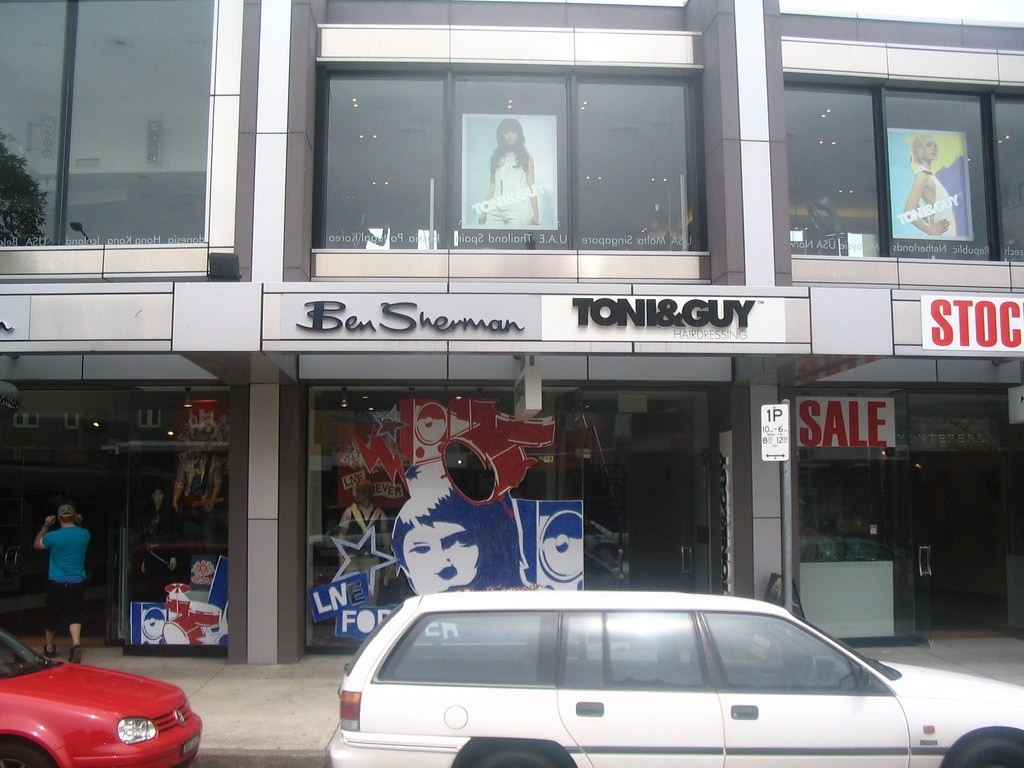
[44,642,56,660]
[68,645,81,663]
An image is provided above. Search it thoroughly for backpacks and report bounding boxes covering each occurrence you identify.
[345,507,381,556]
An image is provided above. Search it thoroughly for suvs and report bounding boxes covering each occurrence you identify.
[327,588,1023,768]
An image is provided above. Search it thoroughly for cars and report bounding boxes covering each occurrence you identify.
[0,626,204,768]
[581,519,632,567]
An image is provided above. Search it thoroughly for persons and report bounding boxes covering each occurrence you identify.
[33,504,91,664]
[140,484,182,604]
[902,133,956,237]
[478,120,540,225]
[338,479,392,608]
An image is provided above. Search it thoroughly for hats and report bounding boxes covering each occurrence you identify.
[58,504,74,518]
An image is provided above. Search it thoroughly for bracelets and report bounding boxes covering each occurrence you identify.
[43,523,49,528]
